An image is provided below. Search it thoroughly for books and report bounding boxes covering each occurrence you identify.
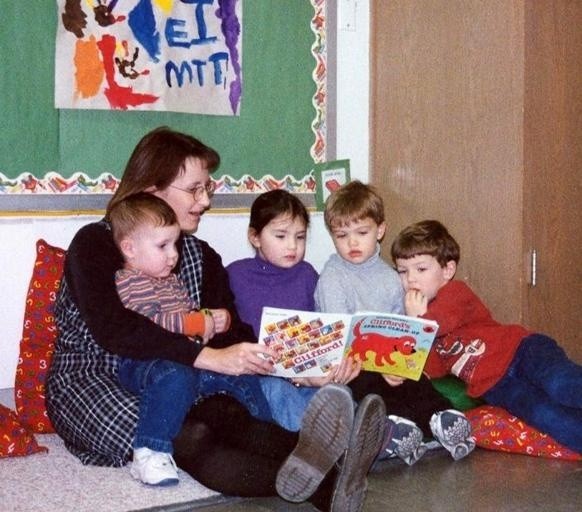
[255,306,440,382]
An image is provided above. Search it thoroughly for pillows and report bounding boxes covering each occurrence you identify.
[1,235,67,463]
[459,404,582,462]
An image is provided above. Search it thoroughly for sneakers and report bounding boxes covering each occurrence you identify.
[271,380,390,512]
[128,444,180,491]
[428,408,479,465]
[389,415,429,470]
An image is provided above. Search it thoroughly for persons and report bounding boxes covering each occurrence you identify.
[45,124,388,512]
[99,189,279,488]
[390,218,581,455]
[222,188,394,462]
[313,177,479,467]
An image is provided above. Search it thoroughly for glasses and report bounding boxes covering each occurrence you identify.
[165,179,218,203]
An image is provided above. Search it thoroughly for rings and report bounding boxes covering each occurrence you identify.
[333,378,340,384]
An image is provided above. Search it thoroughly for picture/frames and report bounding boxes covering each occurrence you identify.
[313,157,351,212]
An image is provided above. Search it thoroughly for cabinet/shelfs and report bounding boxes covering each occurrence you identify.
[368,0,582,361]
